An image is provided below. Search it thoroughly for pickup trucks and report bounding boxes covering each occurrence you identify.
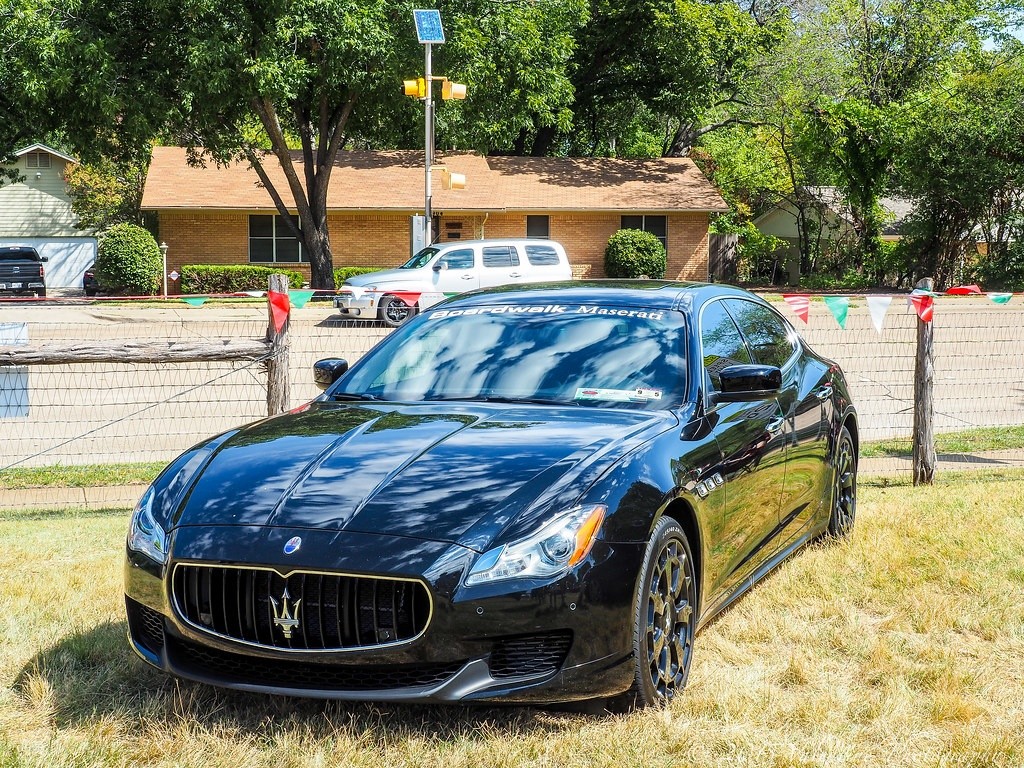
[0,246,49,297]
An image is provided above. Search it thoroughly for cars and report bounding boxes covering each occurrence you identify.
[333,239,573,328]
[83,263,107,296]
[123,278,859,712]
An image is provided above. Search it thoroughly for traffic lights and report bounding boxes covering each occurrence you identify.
[400,78,426,98]
[441,82,466,100]
[442,172,466,190]
